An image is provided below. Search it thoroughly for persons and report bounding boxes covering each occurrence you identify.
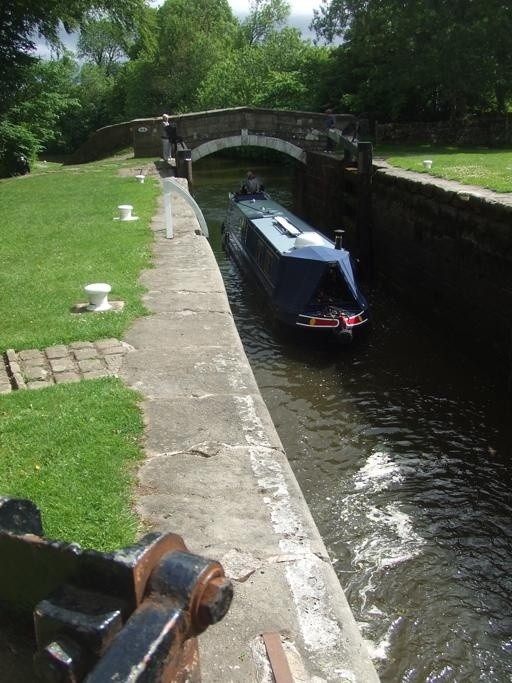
[160,114,171,160]
[241,171,260,194]
[324,109,336,153]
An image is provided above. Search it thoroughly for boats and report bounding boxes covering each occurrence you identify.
[221,179,372,345]
